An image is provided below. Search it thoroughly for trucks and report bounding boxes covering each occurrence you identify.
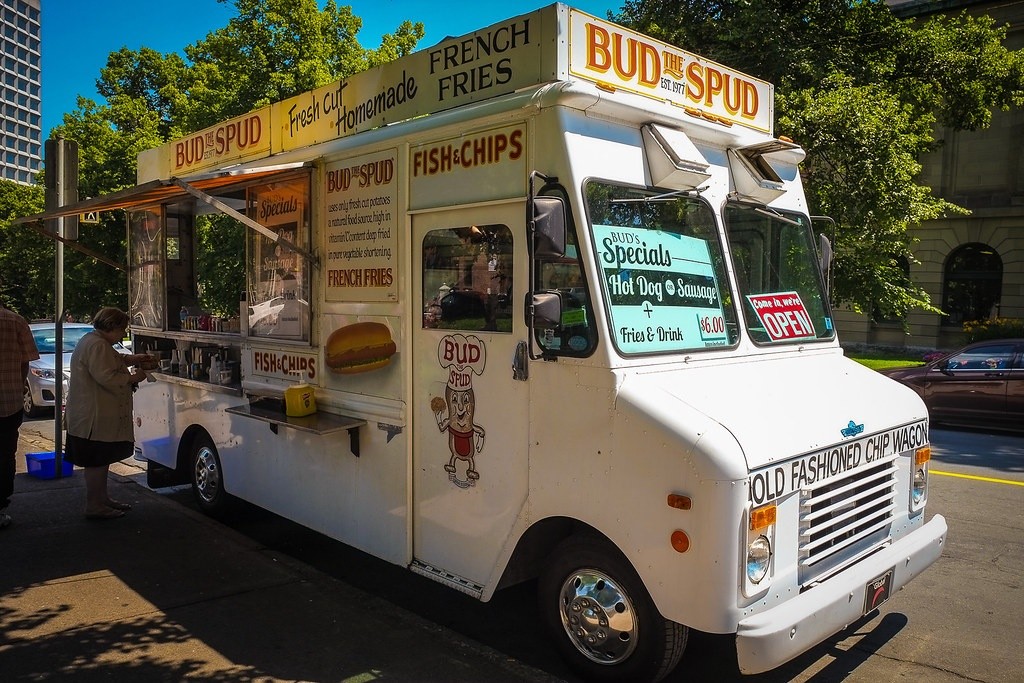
[124,0,947,683]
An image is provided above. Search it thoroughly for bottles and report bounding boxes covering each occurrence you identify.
[179,306,240,333]
[240,291,249,337]
[170,347,231,384]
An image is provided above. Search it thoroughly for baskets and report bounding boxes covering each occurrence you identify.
[25,452,73,479]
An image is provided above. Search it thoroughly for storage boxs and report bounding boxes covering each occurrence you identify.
[24,452,74,479]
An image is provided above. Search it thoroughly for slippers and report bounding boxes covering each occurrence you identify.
[84,505,125,519]
[103,500,131,510]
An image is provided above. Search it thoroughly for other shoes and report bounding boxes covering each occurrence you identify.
[0,514,12,529]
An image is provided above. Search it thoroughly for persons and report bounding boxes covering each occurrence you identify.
[64,308,156,521]
[0,304,41,527]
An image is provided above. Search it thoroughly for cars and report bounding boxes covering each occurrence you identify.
[23,320,132,418]
[558,284,587,306]
[871,337,1024,437]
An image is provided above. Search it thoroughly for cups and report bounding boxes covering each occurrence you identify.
[157,359,170,370]
